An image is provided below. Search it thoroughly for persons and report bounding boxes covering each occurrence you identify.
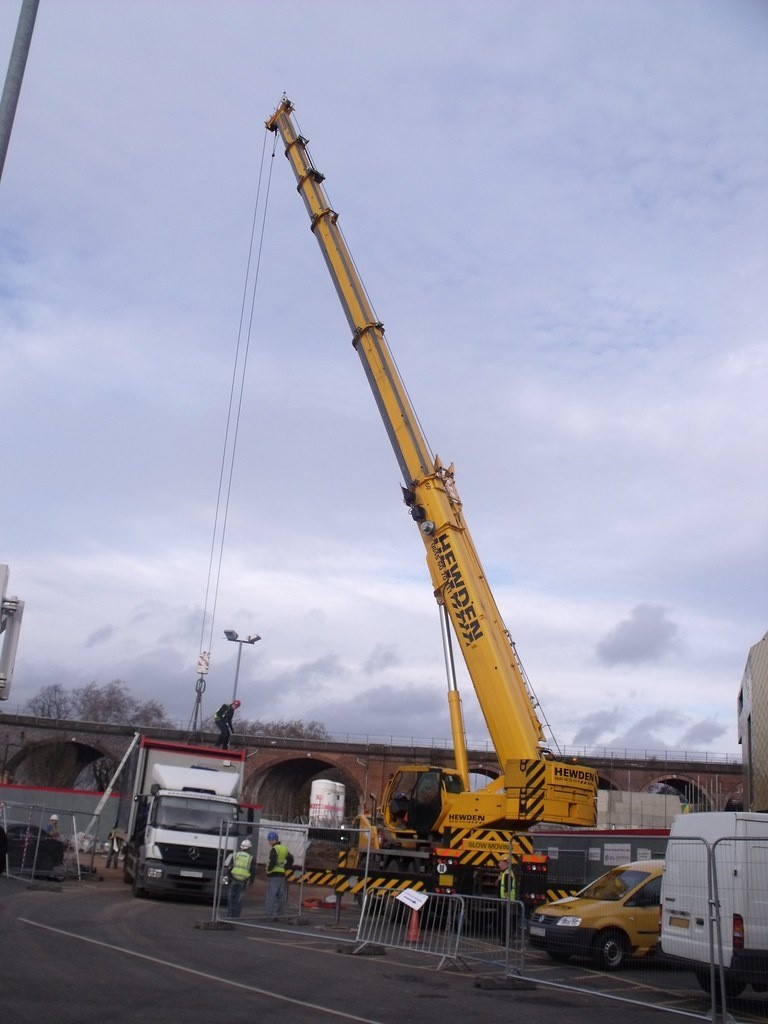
[43,814,59,840]
[213,699,241,750]
[260,831,294,922]
[495,853,515,947]
[105,826,126,868]
[223,839,256,918]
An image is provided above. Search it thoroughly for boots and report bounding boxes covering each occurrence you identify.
[105,860,111,867]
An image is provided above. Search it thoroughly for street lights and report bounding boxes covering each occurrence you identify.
[222,629,262,702]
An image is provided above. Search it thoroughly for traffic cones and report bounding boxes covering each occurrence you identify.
[403,909,425,944]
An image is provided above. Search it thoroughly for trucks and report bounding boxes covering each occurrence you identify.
[112,734,247,905]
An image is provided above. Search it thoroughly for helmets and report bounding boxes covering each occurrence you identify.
[240,840,252,849]
[234,700,241,707]
[268,832,278,840]
[498,854,509,860]
[50,815,59,820]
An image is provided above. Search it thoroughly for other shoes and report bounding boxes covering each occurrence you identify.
[223,744,228,749]
[274,918,282,922]
[215,743,219,747]
[114,861,117,869]
[261,918,274,922]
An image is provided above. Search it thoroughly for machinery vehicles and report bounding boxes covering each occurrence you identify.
[265,98,596,933]
[526,860,666,970]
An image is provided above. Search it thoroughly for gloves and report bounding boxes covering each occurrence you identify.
[231,729,234,734]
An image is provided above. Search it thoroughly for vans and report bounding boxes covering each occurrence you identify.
[659,810,768,998]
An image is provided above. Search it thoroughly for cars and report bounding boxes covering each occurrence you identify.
[0,819,64,866]
